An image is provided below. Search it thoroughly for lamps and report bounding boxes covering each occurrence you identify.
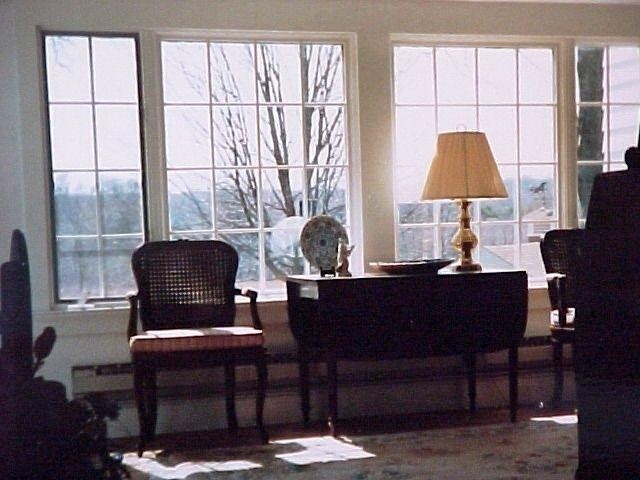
[422,132,506,272]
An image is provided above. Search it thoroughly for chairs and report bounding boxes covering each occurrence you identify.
[128,239,270,459]
[540,229,589,409]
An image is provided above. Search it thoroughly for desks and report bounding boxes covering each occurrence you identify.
[286,270,528,438]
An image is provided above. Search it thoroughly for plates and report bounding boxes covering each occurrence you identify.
[301,214,350,270]
[369,259,454,277]
